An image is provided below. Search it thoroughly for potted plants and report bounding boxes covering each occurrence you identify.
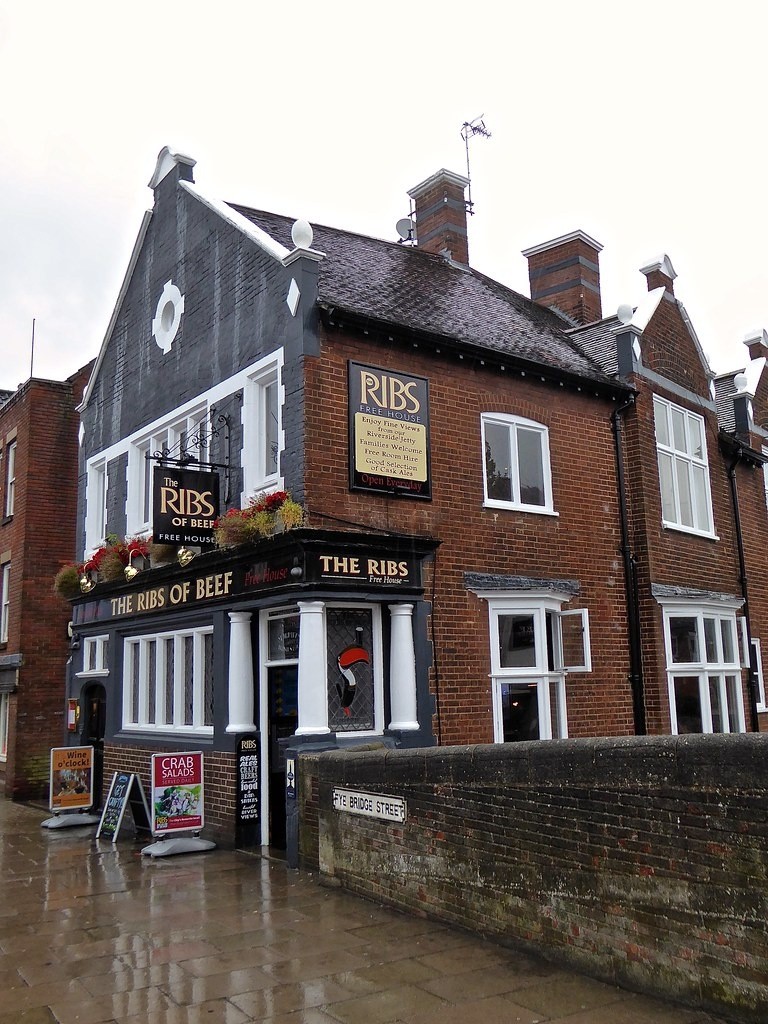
[148,537,178,569]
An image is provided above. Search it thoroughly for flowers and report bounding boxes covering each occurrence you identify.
[91,544,129,565]
[78,560,100,575]
[212,489,304,551]
[123,540,149,558]
[54,560,79,590]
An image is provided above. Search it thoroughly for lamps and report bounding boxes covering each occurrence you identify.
[80,562,100,593]
[123,548,147,582]
[176,545,197,568]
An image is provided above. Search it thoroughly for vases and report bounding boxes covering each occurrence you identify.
[185,546,201,555]
[132,557,150,571]
[254,510,284,539]
[60,581,82,598]
[100,561,123,583]
[80,571,101,583]
